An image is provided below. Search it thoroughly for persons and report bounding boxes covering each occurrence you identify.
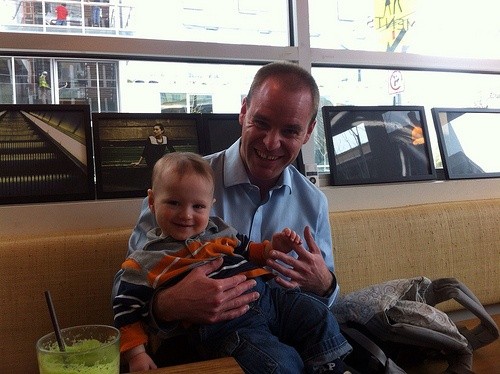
[128,124,177,168]
[91,0,101,25]
[37,71,50,98]
[55,4,70,26]
[112,62,340,374]
[112,153,359,374]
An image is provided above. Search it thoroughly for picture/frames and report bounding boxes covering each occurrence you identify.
[91,112,209,200]
[431,107,500,180]
[321,105,438,186]
[195,113,306,185]
[0,103,96,204]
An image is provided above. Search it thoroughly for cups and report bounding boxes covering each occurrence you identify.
[36,325,120,374]
[305,162,319,187]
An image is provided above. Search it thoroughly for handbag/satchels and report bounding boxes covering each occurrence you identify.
[331,275,500,374]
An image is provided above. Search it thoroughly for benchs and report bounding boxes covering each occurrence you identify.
[0,178,500,374]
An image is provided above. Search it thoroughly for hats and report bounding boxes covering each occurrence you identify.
[42,71,47,74]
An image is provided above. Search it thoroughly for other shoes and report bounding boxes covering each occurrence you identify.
[306,358,360,374]
